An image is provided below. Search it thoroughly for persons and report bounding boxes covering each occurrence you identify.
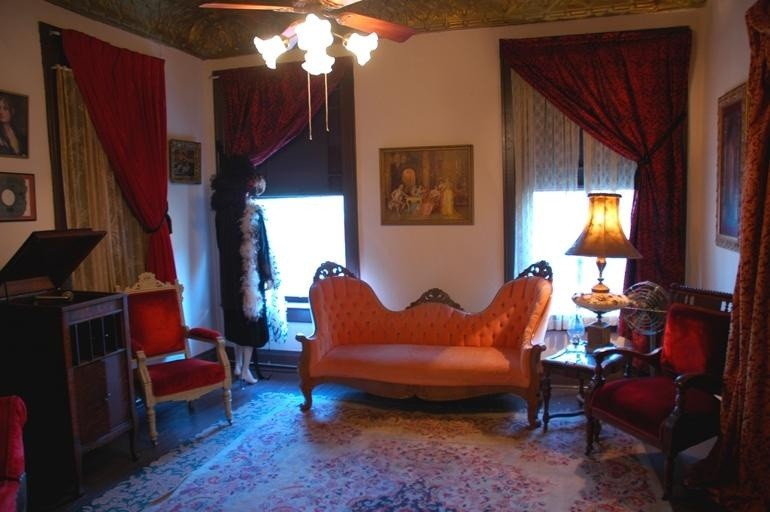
[390,175,457,217]
[0,94,24,155]
[209,152,274,388]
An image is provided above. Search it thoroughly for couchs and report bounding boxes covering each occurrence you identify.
[295,260,554,427]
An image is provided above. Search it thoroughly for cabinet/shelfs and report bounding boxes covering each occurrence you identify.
[0,289,142,496]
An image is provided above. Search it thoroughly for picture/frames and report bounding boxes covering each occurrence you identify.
[379,144,475,226]
[0,88,29,159]
[168,139,202,185]
[0,172,37,222]
[715,82,748,253]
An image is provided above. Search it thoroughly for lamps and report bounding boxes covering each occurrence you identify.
[253,13,379,141]
[564,193,644,353]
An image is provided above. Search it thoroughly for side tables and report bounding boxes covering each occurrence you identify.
[541,340,626,443]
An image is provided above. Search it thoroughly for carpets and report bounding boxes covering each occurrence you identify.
[66,391,671,512]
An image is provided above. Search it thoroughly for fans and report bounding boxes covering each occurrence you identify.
[199,0,418,43]
[621,280,671,377]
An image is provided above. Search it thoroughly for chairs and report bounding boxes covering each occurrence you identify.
[582,302,733,500]
[668,284,733,314]
[0,395,27,512]
[113,272,233,447]
